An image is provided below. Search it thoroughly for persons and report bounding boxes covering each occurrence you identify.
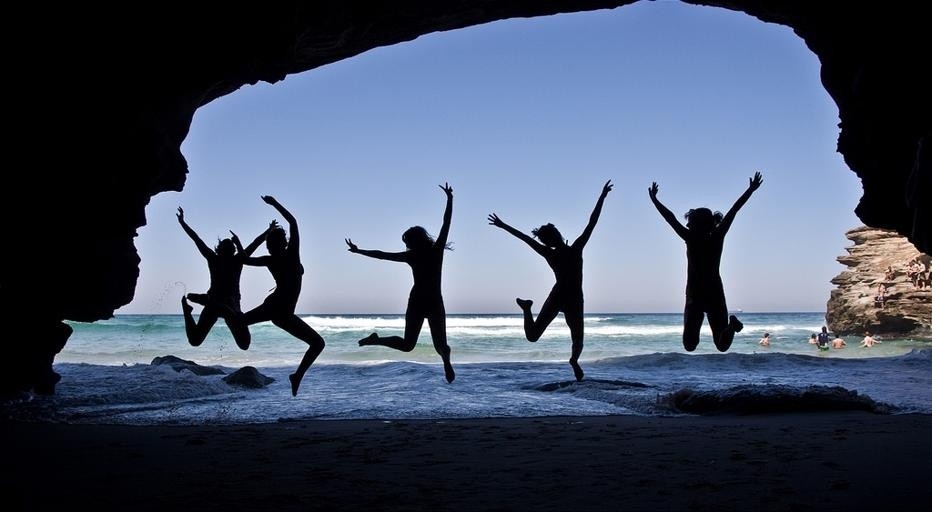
[486,179,615,383]
[644,170,765,352]
[343,182,457,383]
[758,324,883,351]
[187,193,326,397]
[175,204,279,350]
